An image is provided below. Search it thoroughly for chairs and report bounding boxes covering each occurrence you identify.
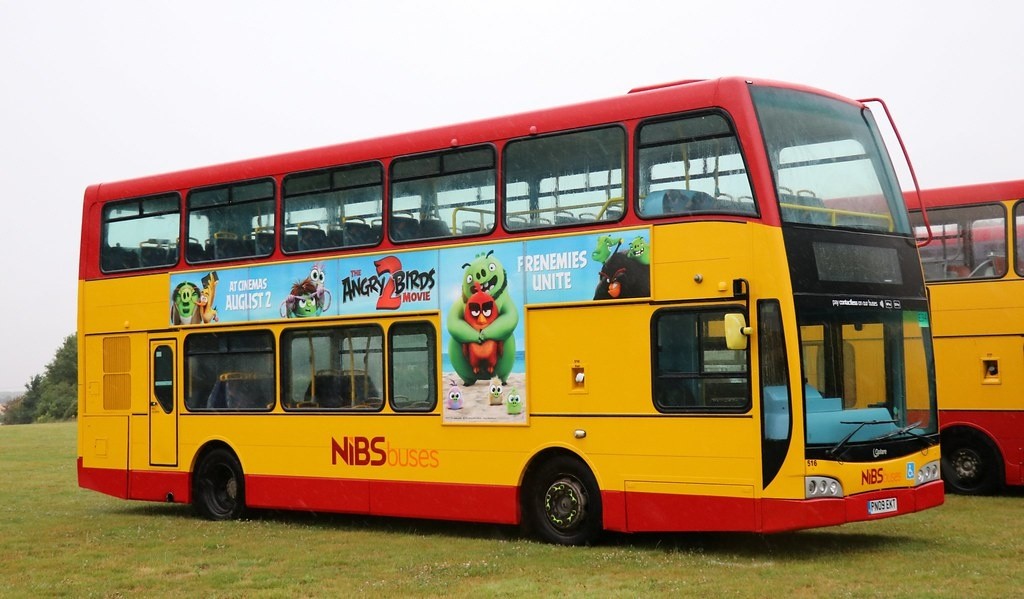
[104,185,828,271]
[942,255,1024,280]
[187,368,433,411]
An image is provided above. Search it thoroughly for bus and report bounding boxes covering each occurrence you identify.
[818,179,1024,499]
[71,74,948,548]
